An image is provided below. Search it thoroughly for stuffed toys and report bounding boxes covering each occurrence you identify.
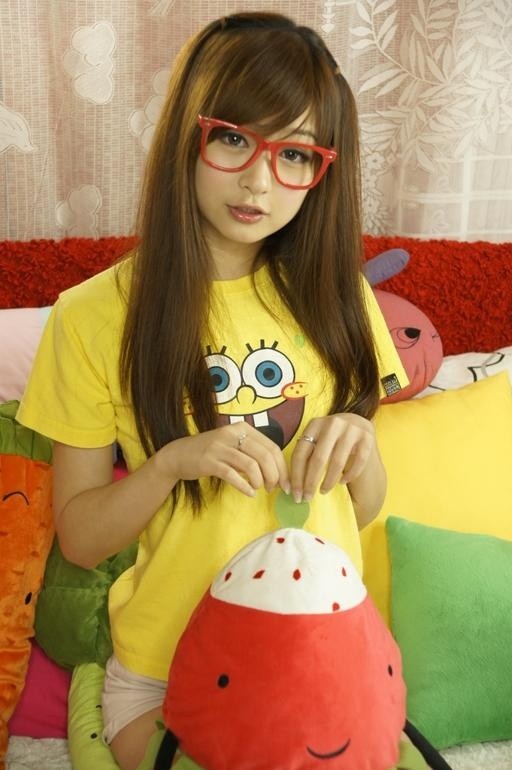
[160,528,406,770]
[361,249,443,404]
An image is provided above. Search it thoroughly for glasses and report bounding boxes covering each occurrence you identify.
[192,109,335,194]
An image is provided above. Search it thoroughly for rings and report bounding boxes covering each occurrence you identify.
[297,435,317,445]
[237,433,249,449]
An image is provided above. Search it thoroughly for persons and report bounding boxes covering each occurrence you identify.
[15,11,411,770]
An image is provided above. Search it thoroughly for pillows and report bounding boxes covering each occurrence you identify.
[414,342,511,399]
[378,513,512,753]
[344,365,512,648]
[1,307,51,400]
